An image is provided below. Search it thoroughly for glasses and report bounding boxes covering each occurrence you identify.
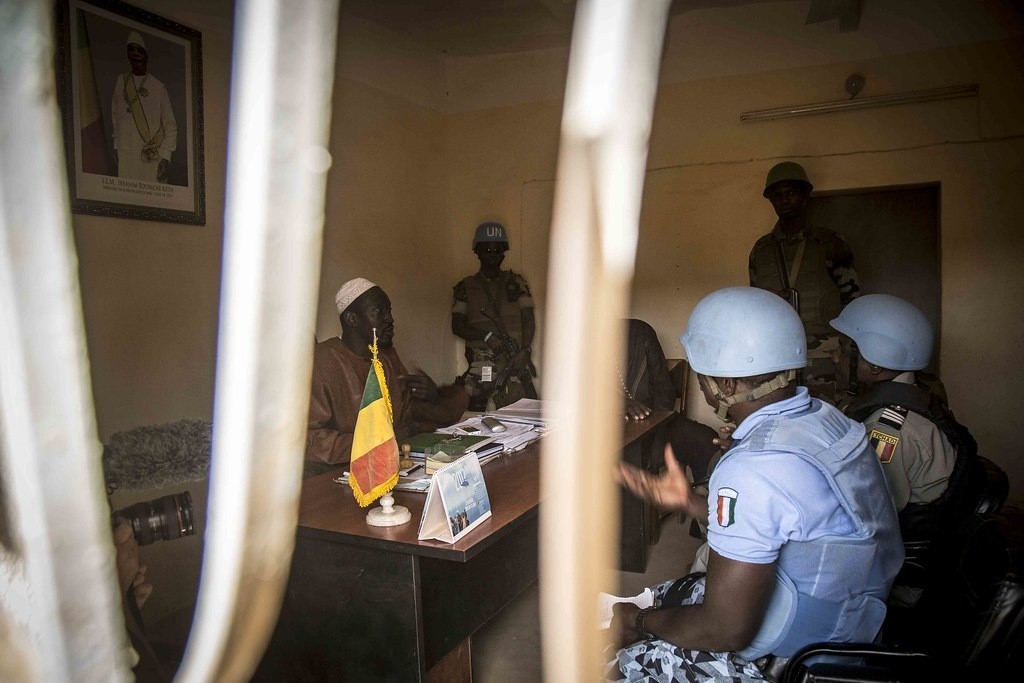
[476,246,505,253]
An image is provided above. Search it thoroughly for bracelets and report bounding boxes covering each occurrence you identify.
[635,606,655,641]
[484,331,493,342]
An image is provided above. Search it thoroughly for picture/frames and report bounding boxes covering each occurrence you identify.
[57,0,204,226]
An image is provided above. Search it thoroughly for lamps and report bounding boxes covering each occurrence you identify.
[739,82,980,123]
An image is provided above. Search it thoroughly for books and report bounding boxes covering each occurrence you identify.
[397,432,504,460]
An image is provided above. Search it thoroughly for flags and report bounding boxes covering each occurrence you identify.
[348,359,400,507]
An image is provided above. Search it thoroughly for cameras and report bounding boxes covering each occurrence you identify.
[110,491,197,547]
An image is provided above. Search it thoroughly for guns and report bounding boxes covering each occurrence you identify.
[479,300,538,402]
[766,238,801,388]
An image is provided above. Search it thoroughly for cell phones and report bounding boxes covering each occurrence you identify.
[457,424,482,435]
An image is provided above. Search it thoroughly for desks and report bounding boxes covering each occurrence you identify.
[251,400,676,683]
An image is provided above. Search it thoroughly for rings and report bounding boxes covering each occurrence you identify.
[412,388,416,392]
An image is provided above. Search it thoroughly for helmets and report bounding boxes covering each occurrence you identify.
[472,222,510,253]
[829,294,933,371]
[682,287,810,378]
[763,160,813,197]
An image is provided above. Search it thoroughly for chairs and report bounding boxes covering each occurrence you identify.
[779,414,1024,683]
[666,358,690,415]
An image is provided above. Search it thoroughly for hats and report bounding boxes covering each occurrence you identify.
[335,278,377,317]
[125,31,147,50]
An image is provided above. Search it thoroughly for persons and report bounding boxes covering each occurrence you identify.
[303,278,450,480]
[602,286,905,683]
[111,32,177,183]
[619,318,720,482]
[749,161,862,409]
[451,222,535,410]
[712,293,959,604]
